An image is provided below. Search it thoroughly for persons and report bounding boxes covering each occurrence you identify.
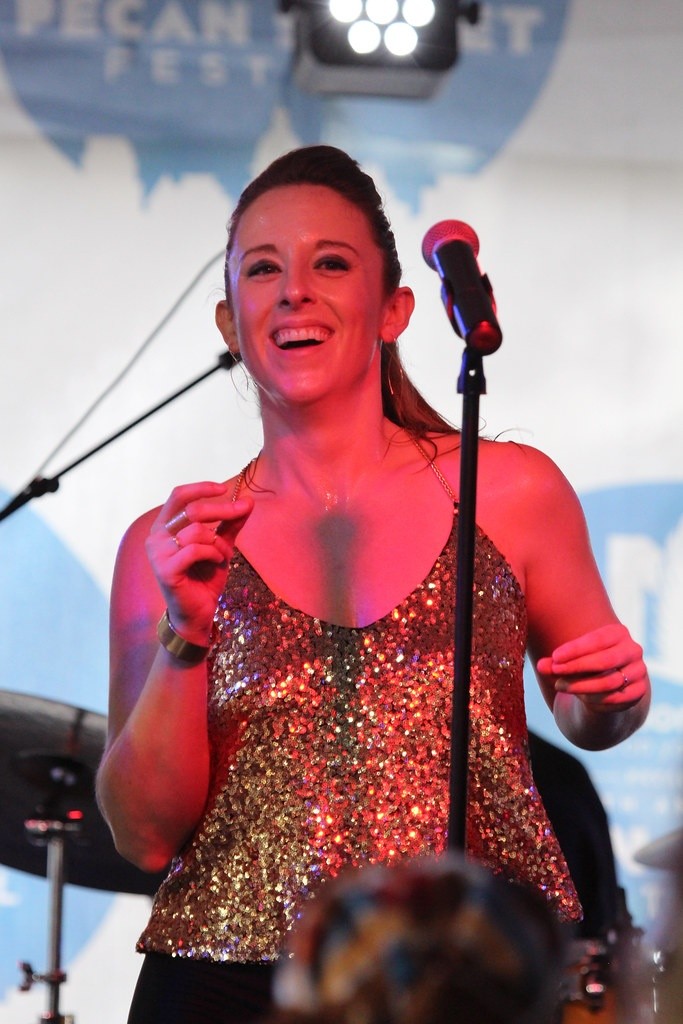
[524,726,631,1024]
[270,853,571,1024]
[93,145,651,1024]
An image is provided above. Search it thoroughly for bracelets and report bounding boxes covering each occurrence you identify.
[156,608,210,662]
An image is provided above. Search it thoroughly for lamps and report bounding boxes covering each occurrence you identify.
[277,1,482,98]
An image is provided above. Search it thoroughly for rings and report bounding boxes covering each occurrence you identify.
[165,511,188,537]
[172,536,183,549]
[616,668,628,684]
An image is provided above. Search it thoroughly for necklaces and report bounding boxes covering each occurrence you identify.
[321,487,357,513]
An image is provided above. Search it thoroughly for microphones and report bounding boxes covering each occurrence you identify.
[421,220,503,355]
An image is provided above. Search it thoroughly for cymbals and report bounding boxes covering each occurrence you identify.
[631,824,683,873]
[1,690,160,897]
